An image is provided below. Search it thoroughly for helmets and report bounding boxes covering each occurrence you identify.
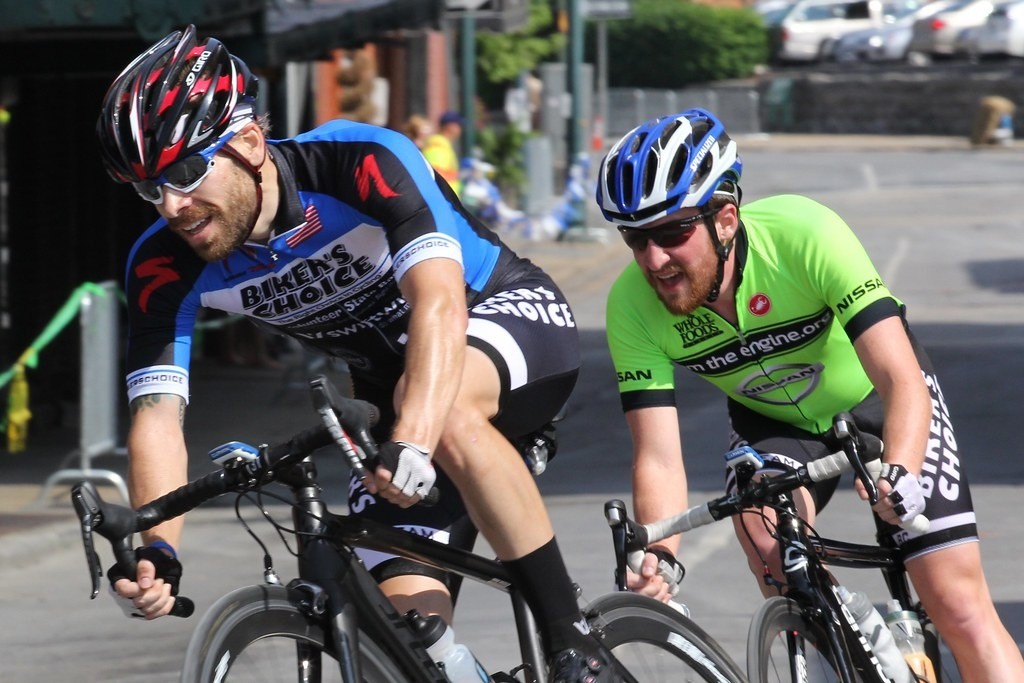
[95,24,259,184]
[596,108,743,227]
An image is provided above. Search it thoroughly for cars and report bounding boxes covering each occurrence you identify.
[752,0,1023,71]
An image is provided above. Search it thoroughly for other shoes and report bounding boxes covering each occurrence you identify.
[548,649,613,683]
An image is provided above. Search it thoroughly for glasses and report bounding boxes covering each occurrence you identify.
[617,207,720,251]
[132,116,254,205]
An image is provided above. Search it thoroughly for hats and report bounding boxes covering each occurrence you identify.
[439,112,464,126]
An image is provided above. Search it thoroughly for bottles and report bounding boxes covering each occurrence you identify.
[884,599,937,683]
[403,607,496,683]
[837,586,919,683]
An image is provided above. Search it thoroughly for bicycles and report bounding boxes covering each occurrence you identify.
[604,411,945,683]
[70,373,748,683]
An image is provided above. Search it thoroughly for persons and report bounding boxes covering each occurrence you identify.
[97,26,613,683]
[405,109,462,198]
[597,108,1024,683]
